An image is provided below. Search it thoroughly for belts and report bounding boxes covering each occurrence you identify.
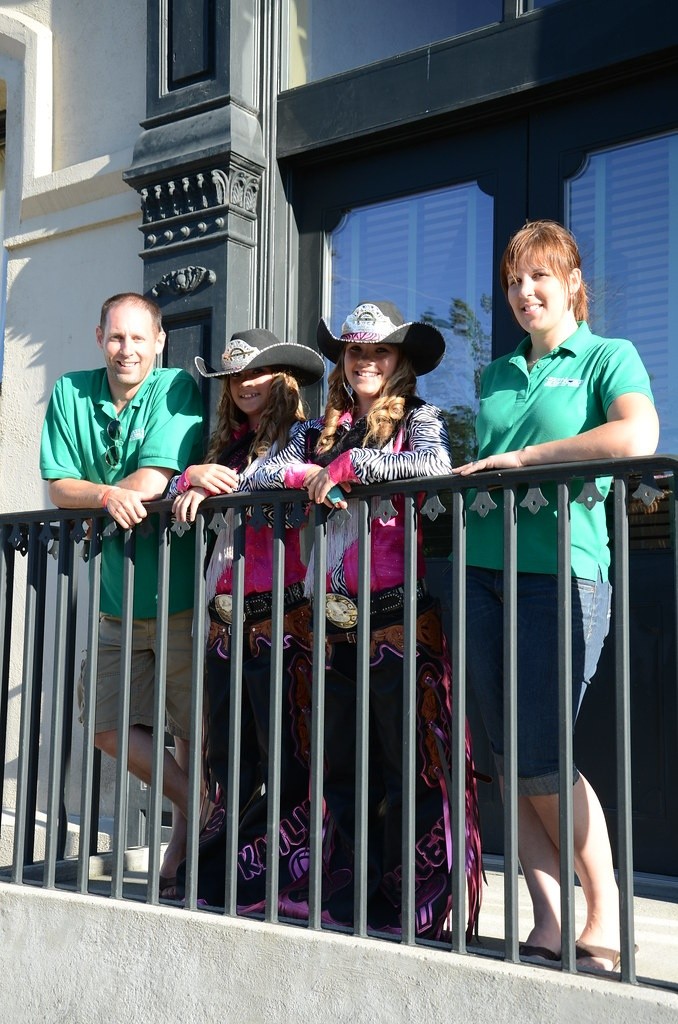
[325,577,433,634]
[209,580,310,627]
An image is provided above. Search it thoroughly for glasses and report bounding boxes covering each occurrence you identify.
[104,418,122,469]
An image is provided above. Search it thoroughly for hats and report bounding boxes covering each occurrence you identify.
[193,329,326,388]
[316,301,446,376]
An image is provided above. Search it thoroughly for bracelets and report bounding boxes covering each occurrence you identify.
[102,486,114,509]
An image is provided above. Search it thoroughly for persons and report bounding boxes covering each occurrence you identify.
[170,301,484,944]
[445,222,660,973]
[37,292,220,896]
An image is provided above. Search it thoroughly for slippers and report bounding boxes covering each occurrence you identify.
[519,945,561,961]
[576,939,639,972]
[159,875,178,900]
